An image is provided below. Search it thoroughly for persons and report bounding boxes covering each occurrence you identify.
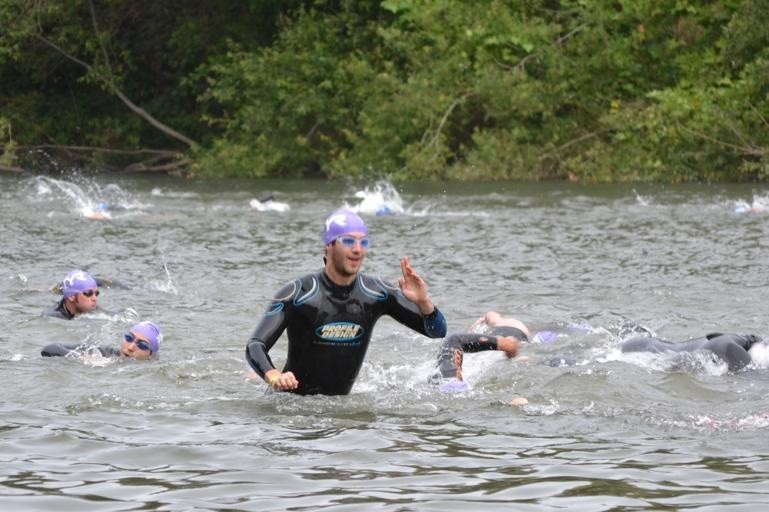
[621,330,762,375]
[470,311,559,350]
[41,322,164,362]
[43,270,111,321]
[245,207,447,395]
[436,334,518,397]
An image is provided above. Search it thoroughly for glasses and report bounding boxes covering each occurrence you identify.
[124,332,154,351]
[82,290,100,298]
[338,235,369,248]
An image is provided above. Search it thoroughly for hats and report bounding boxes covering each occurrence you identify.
[323,208,369,245]
[62,269,97,298]
[131,321,162,352]
